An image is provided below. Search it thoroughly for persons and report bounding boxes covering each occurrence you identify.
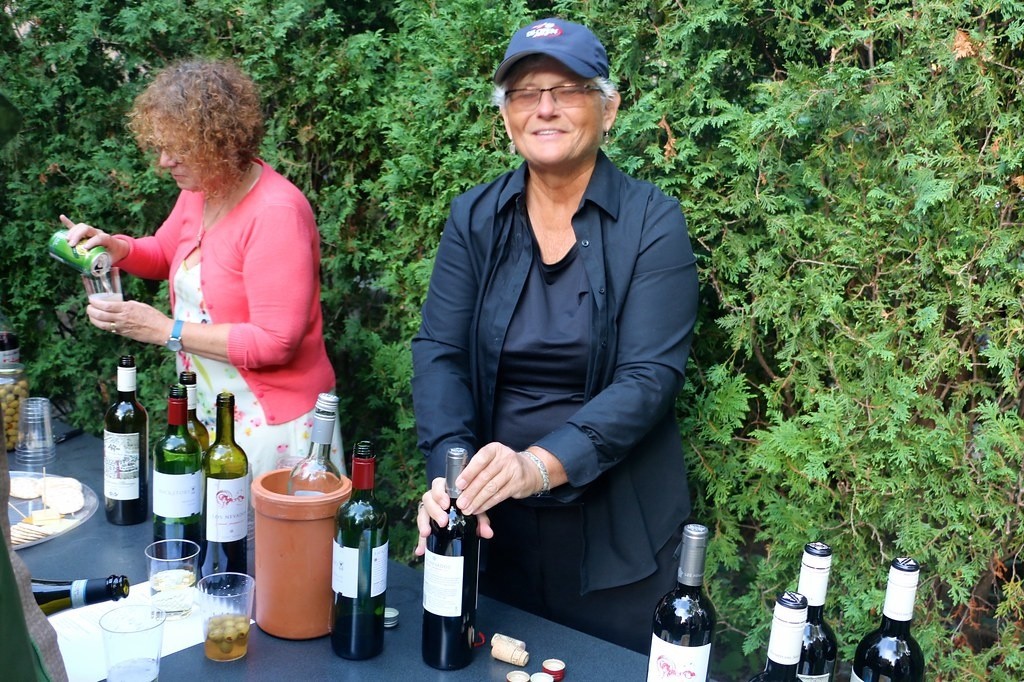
[60,53,348,545]
[410,17,701,653]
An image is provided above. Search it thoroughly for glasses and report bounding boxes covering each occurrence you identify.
[504,83,604,111]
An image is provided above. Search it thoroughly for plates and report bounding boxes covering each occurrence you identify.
[8,470,100,551]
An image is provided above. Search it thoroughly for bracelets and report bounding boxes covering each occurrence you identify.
[523,451,549,497]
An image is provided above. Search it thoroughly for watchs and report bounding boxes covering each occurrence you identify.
[165,320,184,351]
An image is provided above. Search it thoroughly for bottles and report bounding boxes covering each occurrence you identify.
[793,542,837,682]
[331,441,388,661]
[0,308,20,362]
[422,447,480,669]
[645,523,717,681]
[287,393,339,496]
[174,372,209,455]
[103,355,150,526]
[152,384,206,571]
[197,394,249,585]
[0,363,30,452]
[849,553,926,682]
[751,590,809,681]
[31,574,130,616]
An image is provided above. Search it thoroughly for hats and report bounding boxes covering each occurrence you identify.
[492,19,613,81]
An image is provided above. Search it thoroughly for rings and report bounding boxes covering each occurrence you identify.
[110,322,116,335]
[418,503,424,511]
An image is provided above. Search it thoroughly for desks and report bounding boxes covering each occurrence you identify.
[7,422,649,682]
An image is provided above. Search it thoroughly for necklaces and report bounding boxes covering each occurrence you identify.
[196,163,252,242]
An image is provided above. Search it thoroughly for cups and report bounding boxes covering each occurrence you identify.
[197,573,254,662]
[147,541,200,617]
[248,468,353,638]
[80,267,124,303]
[15,398,58,467]
[99,604,166,681]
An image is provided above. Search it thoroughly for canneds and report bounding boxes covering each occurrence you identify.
[0,363,31,450]
[48,229,112,278]
